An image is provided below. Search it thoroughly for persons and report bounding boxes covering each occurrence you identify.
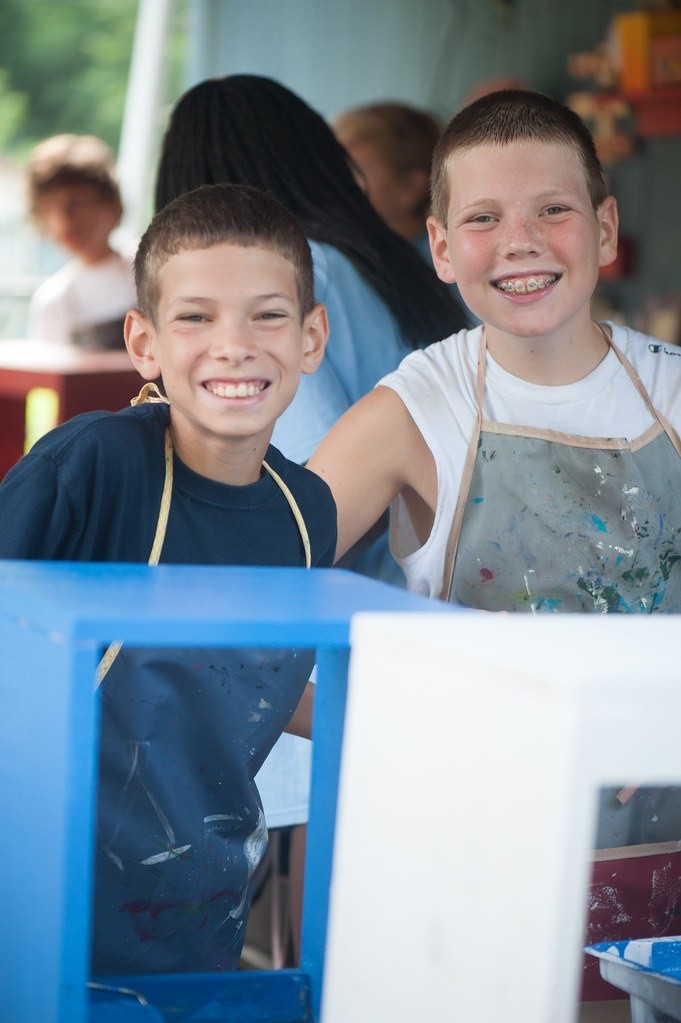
[0,181,338,980]
[331,102,442,242]
[25,132,140,353]
[154,74,484,589]
[305,90,681,1002]
